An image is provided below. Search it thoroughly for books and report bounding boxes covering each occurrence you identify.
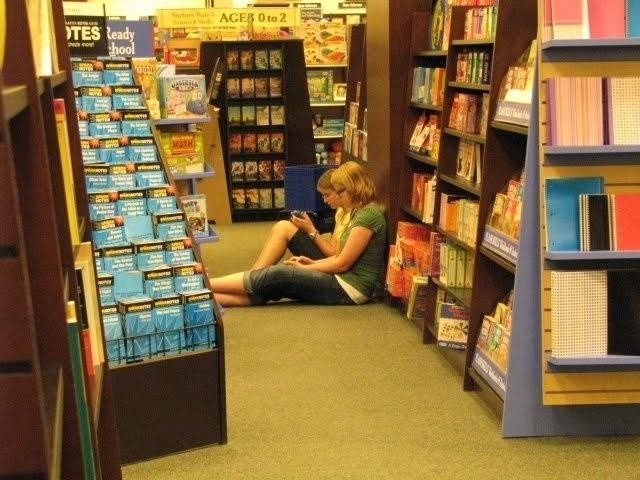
[283,163,337,214]
[338,80,371,162]
[219,47,287,211]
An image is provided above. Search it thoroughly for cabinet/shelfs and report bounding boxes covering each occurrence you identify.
[200,2,369,223]
[393,1,640,439]
[1,0,227,480]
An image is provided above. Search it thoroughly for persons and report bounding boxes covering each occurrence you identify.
[203,161,389,310]
[248,168,352,268]
